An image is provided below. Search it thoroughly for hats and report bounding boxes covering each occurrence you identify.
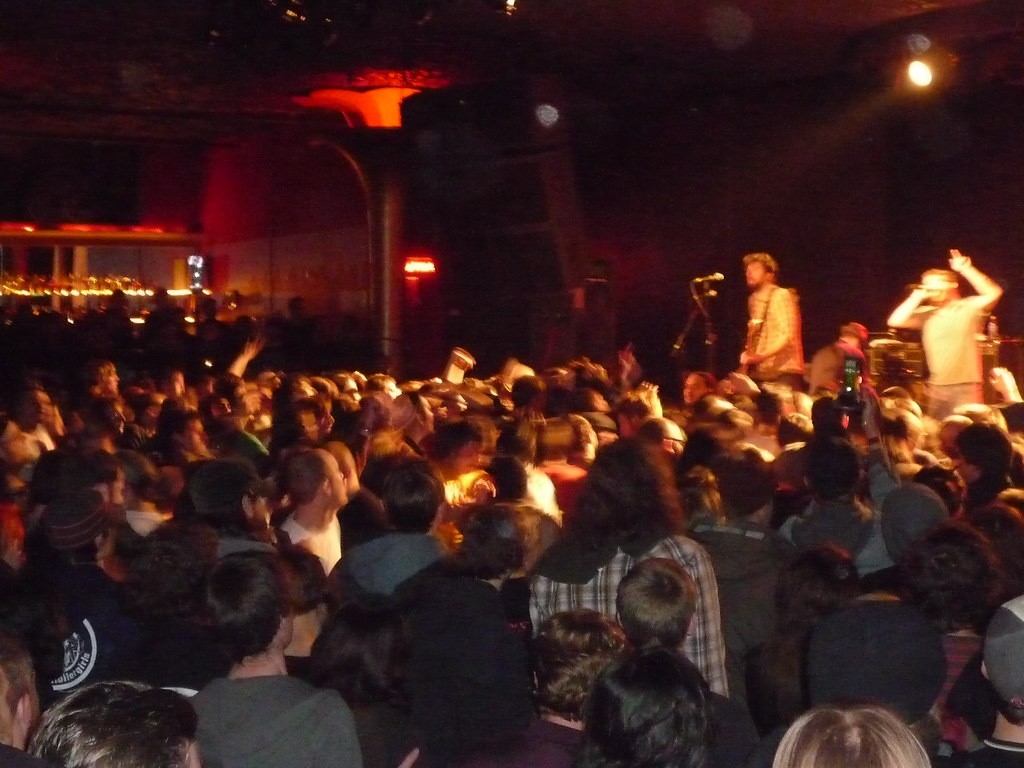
[189,459,282,515]
[41,489,107,550]
[983,595,1024,709]
[743,252,776,272]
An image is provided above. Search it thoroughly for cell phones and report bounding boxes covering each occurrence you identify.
[839,357,862,408]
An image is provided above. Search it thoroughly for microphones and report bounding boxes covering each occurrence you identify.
[694,273,724,283]
[909,284,922,290]
[703,282,718,297]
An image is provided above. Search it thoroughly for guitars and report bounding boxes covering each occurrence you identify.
[739,319,761,376]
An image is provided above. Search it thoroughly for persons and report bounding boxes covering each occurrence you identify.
[0,279,1024,768]
[740,253,804,414]
[887,249,1004,420]
